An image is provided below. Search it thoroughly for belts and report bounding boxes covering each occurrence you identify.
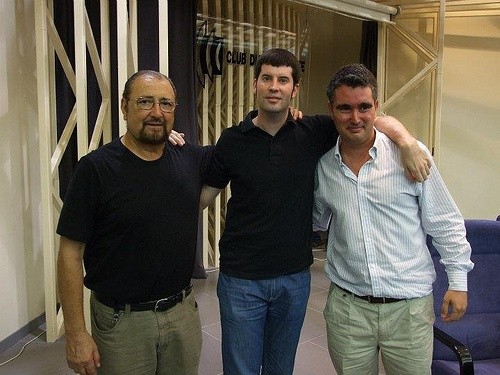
[94,285,194,312]
[334,283,406,304]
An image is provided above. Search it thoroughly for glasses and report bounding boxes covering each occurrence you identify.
[127,96,178,112]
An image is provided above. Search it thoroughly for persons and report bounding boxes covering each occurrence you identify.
[54,69,304,375]
[289,63,474,374]
[165,48,433,375]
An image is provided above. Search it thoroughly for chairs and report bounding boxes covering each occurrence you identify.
[430,216,500,375]
